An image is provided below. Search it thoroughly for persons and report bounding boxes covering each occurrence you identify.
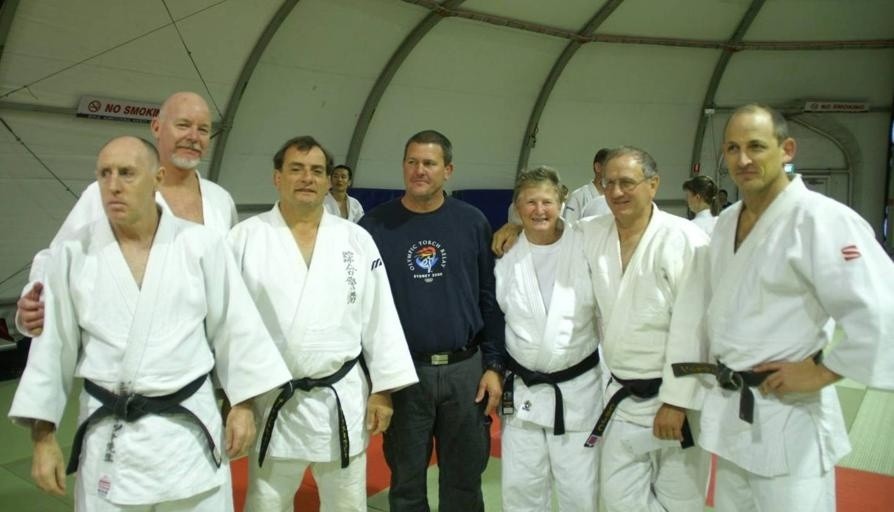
[719,190,732,208]
[681,174,722,237]
[356,129,510,512]
[694,101,893,512]
[13,88,239,339]
[226,135,422,512]
[563,147,613,225]
[492,162,605,512]
[489,143,709,512]
[5,132,292,511]
[322,164,365,223]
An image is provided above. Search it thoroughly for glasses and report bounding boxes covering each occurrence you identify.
[602,176,648,190]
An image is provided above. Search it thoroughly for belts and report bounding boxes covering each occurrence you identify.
[409,345,476,366]
